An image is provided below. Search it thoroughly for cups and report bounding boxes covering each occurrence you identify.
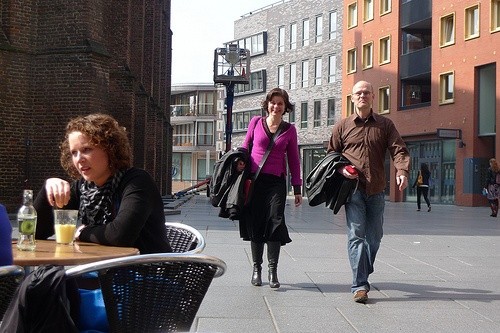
[53,209,80,245]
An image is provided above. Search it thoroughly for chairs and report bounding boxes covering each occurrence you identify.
[0,222,227,333]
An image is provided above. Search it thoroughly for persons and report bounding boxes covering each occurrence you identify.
[33,113,173,256]
[326,80,411,302]
[412,164,431,212]
[233,88,303,288]
[480,158,500,217]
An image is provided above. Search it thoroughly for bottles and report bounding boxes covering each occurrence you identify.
[17,190,37,250]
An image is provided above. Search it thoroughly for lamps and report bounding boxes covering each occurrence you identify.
[458,141,465,148]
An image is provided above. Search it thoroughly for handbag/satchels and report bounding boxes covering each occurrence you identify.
[243,178,254,205]
[416,170,423,185]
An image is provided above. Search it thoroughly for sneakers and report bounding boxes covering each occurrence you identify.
[354,291,367,301]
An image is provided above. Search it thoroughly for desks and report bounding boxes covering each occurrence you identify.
[11,240,141,278]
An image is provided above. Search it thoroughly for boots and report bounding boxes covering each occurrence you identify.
[251,241,264,286]
[267,240,280,288]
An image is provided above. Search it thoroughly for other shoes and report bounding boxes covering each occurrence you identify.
[416,210,420,211]
[490,214,497,217]
[428,207,431,212]
